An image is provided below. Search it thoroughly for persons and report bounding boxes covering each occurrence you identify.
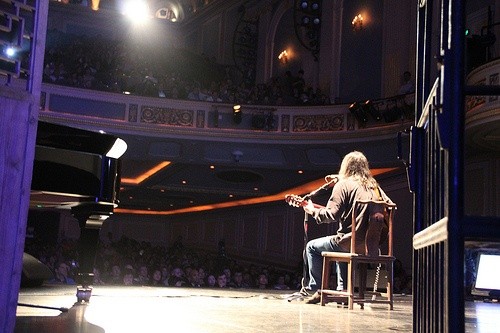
[304,152,395,305]
[0,33,415,132]
[24,225,411,293]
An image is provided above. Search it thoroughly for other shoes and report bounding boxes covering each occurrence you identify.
[305,289,322,303]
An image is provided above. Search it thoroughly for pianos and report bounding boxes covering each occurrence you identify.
[29,121,128,302]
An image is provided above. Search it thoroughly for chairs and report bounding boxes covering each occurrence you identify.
[319,200,396,310]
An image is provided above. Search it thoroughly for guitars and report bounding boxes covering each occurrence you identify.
[285,193,389,245]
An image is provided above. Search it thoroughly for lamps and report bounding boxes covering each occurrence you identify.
[300,0,320,25]
[351,13,362,34]
[278,50,288,65]
